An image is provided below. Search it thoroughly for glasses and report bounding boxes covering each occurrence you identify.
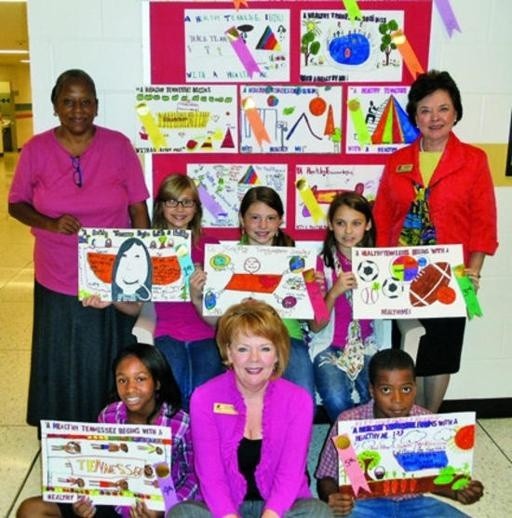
[72,156,81,187]
[164,199,196,207]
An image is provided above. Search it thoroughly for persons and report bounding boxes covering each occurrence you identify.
[316,192,478,428]
[314,347,484,518]
[83,172,227,415]
[167,299,337,518]
[370,69,499,414]
[7,69,152,441]
[189,187,325,403]
[16,343,199,518]
